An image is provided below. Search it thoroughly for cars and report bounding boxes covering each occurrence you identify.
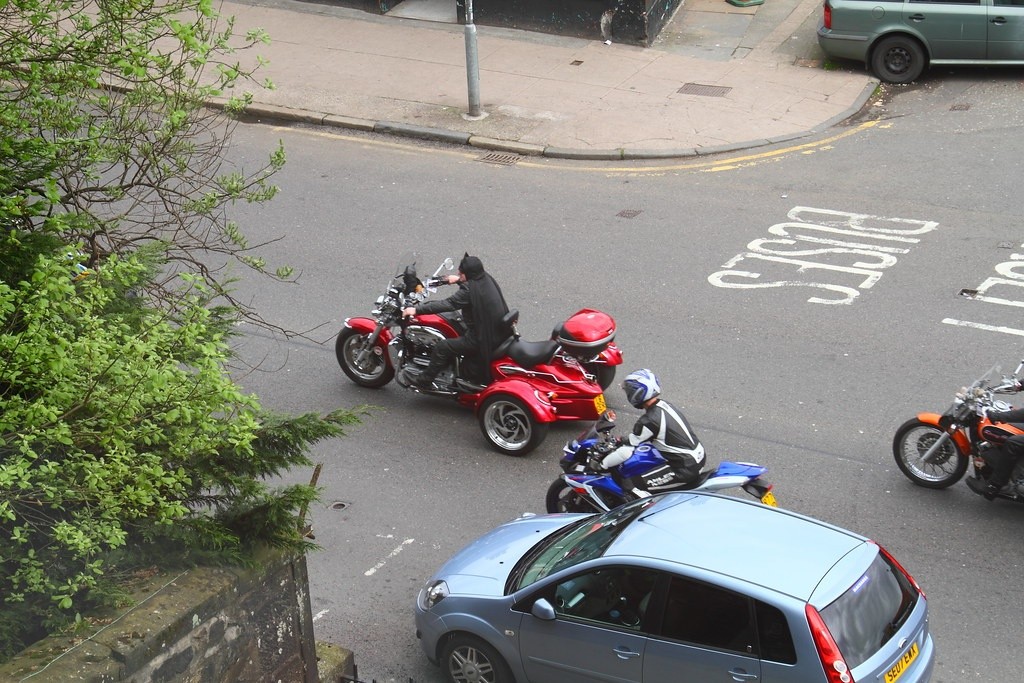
[413,489,936,683]
[816,0,1024,84]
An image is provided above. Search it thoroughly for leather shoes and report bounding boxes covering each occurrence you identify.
[402,369,432,389]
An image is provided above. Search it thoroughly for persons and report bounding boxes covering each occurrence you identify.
[401,252,514,393]
[587,367,706,500]
[964,377,1024,502]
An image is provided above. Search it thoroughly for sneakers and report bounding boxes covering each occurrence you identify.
[966,476,1002,501]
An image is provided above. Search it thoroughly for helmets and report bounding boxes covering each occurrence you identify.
[622,368,662,409]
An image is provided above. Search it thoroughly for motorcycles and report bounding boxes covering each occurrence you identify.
[335,256,625,457]
[545,409,777,514]
[891,360,1024,504]
